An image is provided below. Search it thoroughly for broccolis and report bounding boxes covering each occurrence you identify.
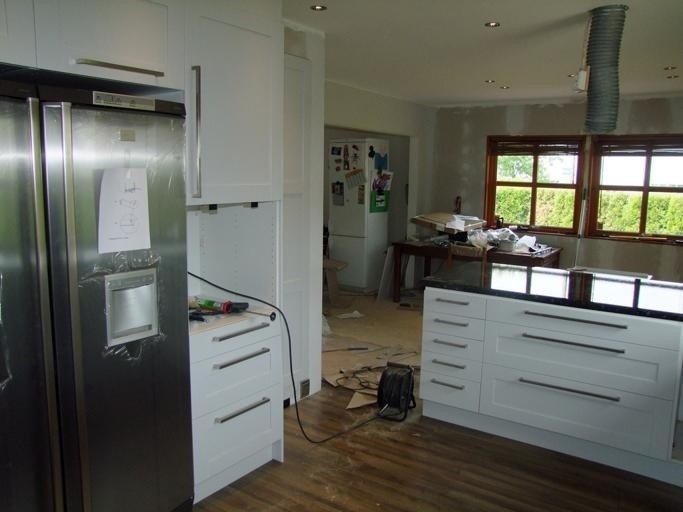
[1,75,197,512]
[327,138,390,295]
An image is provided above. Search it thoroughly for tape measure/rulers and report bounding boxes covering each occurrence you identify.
[189,311,215,322]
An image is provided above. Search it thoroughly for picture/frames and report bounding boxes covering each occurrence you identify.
[389,230,564,310]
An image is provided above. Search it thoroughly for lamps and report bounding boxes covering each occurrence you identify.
[448,243,487,288]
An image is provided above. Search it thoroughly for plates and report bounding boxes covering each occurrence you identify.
[189,308,284,505]
[419,285,683,488]
[184,12,282,206]
[0,0,183,89]
